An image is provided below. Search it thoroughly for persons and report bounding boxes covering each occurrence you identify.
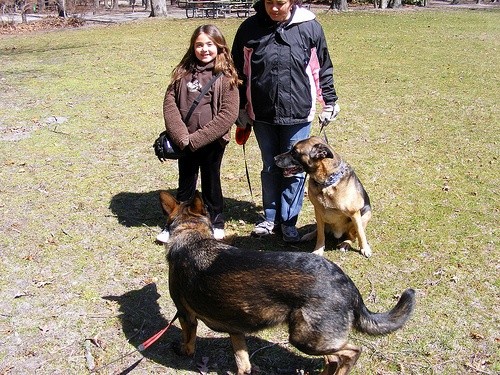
[231,0,341,244]
[155,23,243,244]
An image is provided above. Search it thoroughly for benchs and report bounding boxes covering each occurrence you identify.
[176,0,254,19]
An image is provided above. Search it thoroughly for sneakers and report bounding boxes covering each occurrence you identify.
[156,229,169,243]
[214,228,225,240]
[251,221,274,235]
[282,224,300,242]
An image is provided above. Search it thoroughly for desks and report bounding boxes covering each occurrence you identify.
[188,1,252,18]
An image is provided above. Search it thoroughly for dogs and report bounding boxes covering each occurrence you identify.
[159,190,416,375]
[274,136,372,258]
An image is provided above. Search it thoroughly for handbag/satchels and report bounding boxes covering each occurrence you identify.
[154,130,179,162]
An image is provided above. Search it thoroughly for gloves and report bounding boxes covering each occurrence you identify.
[235,109,254,145]
[318,105,341,126]
